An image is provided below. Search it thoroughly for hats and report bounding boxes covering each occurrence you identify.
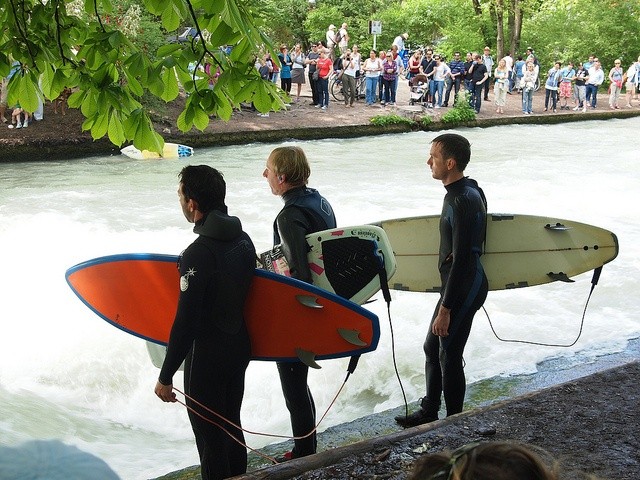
[483,46,491,52]
[328,24,336,30]
[403,34,409,40]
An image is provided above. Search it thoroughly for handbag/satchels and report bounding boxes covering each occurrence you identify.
[312,71,319,80]
[557,82,560,87]
[336,69,343,80]
[334,43,337,49]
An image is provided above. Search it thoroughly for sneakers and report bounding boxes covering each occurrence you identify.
[573,107,579,110]
[366,102,373,107]
[501,110,504,113]
[322,105,328,110]
[616,105,620,109]
[545,108,547,111]
[394,396,443,426]
[346,103,349,107]
[297,98,299,102]
[554,109,558,112]
[442,101,447,107]
[381,101,385,104]
[496,109,499,113]
[565,106,570,110]
[530,112,534,116]
[272,448,297,464]
[610,104,614,110]
[372,100,376,103]
[582,108,587,112]
[351,104,354,107]
[390,101,393,105]
[523,112,528,115]
[315,104,321,107]
[560,106,565,110]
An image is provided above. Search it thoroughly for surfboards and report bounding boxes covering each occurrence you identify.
[145,226,396,370]
[120,142,194,161]
[65,253,382,362]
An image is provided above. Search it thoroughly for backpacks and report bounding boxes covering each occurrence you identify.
[336,31,340,42]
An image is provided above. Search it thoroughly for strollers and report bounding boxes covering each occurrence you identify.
[408,75,430,107]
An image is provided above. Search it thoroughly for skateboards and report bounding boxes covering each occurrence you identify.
[364,213,619,294]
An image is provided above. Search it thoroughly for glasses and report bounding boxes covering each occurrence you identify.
[454,55,459,57]
[319,53,322,56]
[388,56,391,57]
[593,61,597,62]
[311,46,316,48]
[615,63,621,65]
[426,54,432,56]
[527,66,532,69]
[283,48,287,50]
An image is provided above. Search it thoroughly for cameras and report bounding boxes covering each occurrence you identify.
[347,52,351,57]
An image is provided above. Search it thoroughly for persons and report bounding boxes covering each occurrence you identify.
[339,49,356,107]
[305,44,320,106]
[623,56,640,108]
[544,62,561,112]
[572,63,589,113]
[262,147,336,463]
[472,52,478,60]
[326,23,337,62]
[494,59,508,113]
[318,41,327,49]
[583,56,594,106]
[526,47,540,89]
[405,50,422,101]
[501,51,514,95]
[419,49,437,107]
[523,62,537,114]
[154,164,258,480]
[469,55,488,114]
[290,44,305,98]
[432,55,451,107]
[313,49,333,109]
[278,45,293,93]
[383,53,398,105]
[522,54,538,114]
[1,71,71,129]
[590,58,602,70]
[338,22,350,55]
[464,53,475,108]
[391,46,403,103]
[391,33,409,59]
[443,52,465,108]
[394,133,489,427]
[481,46,494,101]
[350,47,361,97]
[378,51,387,104]
[608,58,623,110]
[256,50,273,78]
[256,56,270,117]
[205,63,220,119]
[558,63,575,109]
[362,50,382,106]
[516,56,526,93]
[572,63,605,109]
[270,49,278,83]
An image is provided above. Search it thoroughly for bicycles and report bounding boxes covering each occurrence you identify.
[331,72,366,101]
[507,65,541,92]
